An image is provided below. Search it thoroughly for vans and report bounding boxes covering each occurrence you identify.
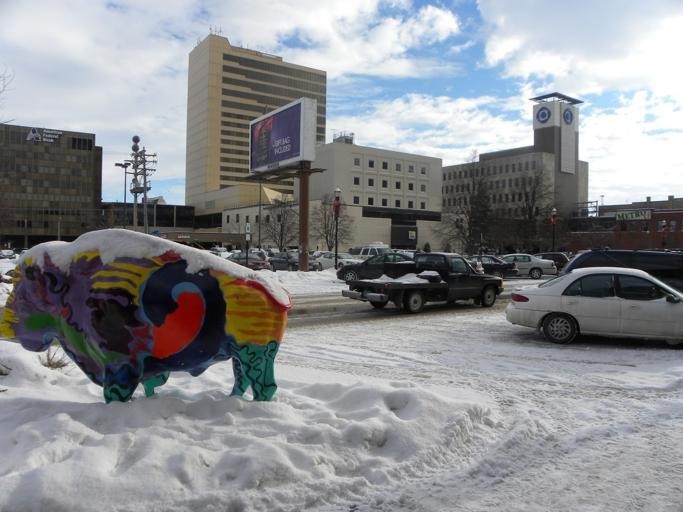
[556,245,682,290]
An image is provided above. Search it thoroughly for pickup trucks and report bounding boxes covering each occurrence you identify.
[339,250,505,314]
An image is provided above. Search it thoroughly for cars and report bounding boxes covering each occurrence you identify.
[0,247,28,261]
[504,265,682,345]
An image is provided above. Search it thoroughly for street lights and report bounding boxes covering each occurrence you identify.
[130,133,141,231]
[332,184,342,272]
[550,207,557,252]
[661,219,668,248]
[113,161,129,229]
